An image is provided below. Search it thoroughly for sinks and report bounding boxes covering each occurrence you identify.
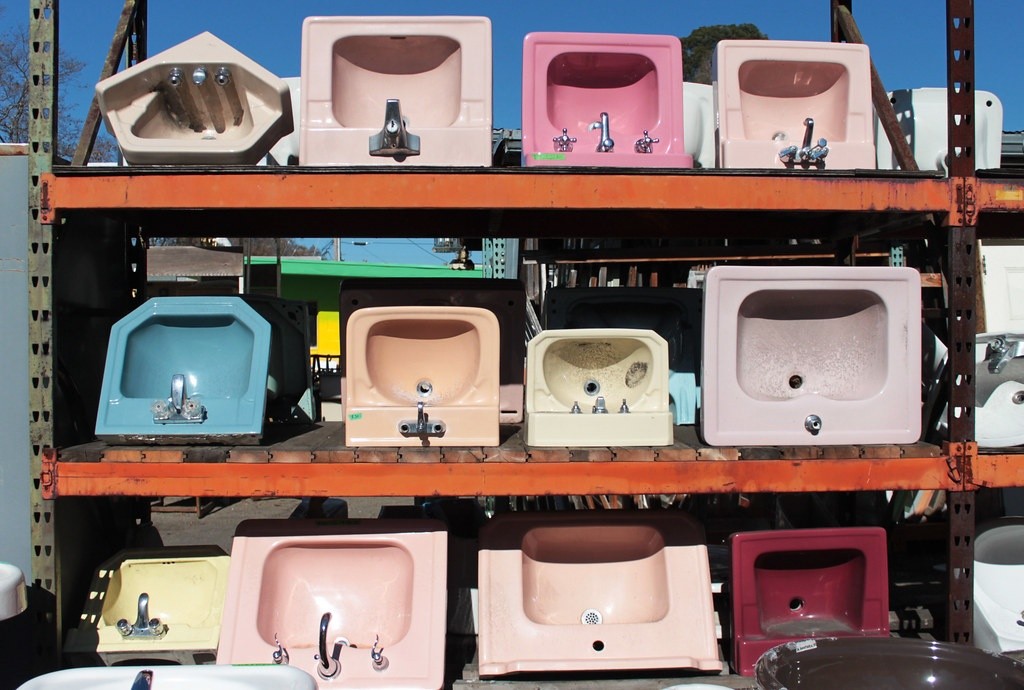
[92,16,1002,171]
[8,508,1024,690]
[95,246,1024,448]
[13,660,319,690]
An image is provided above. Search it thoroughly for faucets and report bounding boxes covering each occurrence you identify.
[192,65,206,88]
[318,614,341,682]
[986,339,1019,374]
[136,593,150,629]
[593,397,607,413]
[417,401,425,431]
[586,112,614,153]
[803,117,814,163]
[369,98,421,155]
[171,374,186,410]
[127,669,157,690]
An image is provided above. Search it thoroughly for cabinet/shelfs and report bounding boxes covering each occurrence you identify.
[26,0,1024,690]
